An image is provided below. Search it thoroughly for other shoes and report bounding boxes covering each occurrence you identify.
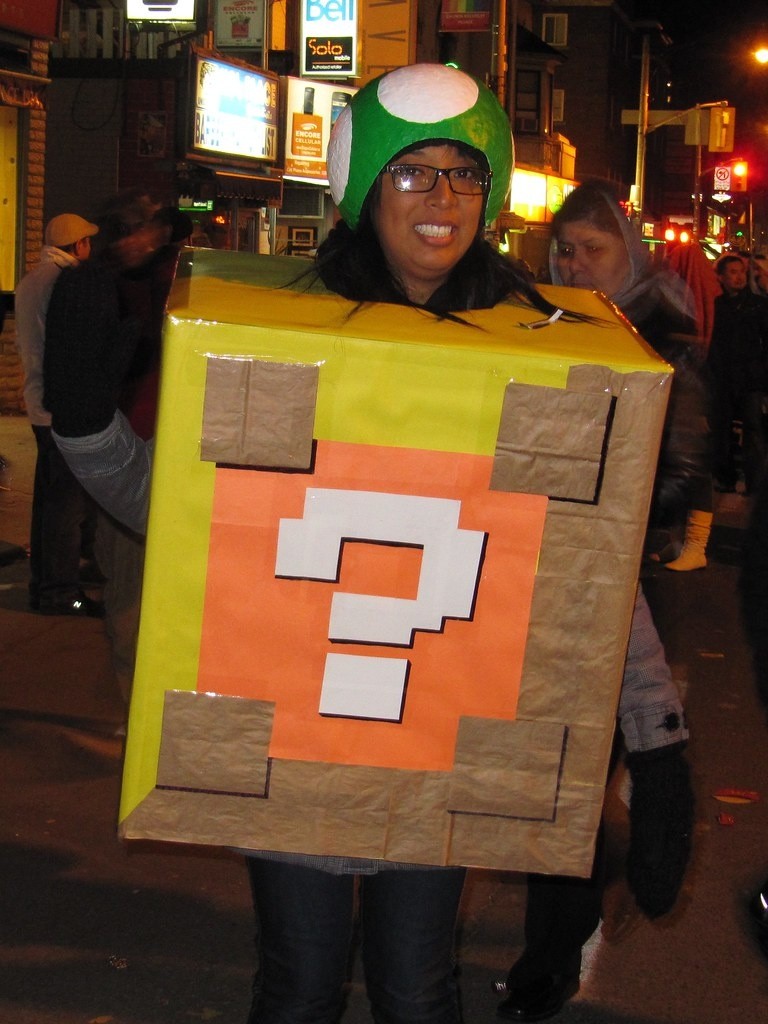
[40,590,107,619]
[81,563,107,587]
[497,974,580,1020]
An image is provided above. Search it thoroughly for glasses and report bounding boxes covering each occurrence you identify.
[381,165,492,195]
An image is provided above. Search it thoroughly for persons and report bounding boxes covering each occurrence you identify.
[495,178,768,1024]
[15,191,190,618]
[44,61,698,1024]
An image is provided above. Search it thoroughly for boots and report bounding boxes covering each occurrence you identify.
[663,510,713,572]
[650,524,683,561]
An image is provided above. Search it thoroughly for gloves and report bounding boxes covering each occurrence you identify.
[624,741,693,919]
[43,260,145,436]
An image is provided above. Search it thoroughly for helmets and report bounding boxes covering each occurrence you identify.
[326,64,515,228]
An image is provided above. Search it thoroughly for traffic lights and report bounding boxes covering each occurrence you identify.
[730,161,747,192]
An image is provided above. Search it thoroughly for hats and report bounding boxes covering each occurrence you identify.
[91,189,192,241]
[45,213,99,246]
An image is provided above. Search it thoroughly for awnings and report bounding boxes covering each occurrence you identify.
[185,168,282,199]
